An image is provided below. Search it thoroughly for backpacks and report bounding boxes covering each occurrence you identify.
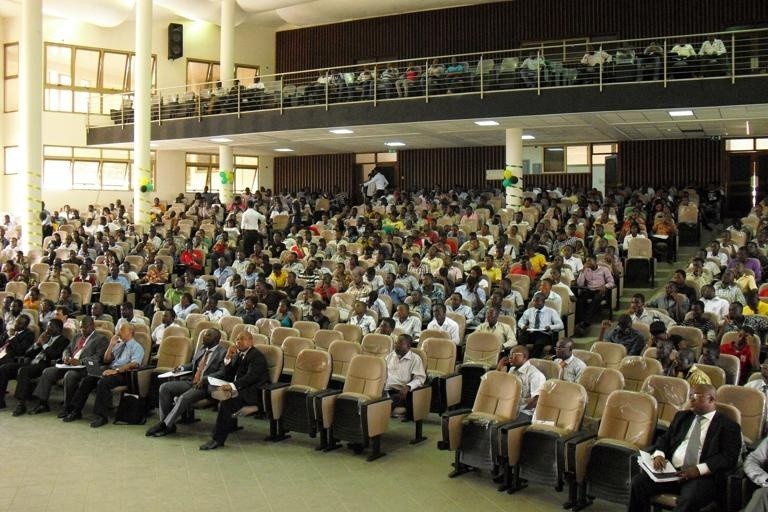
[116,392,147,426]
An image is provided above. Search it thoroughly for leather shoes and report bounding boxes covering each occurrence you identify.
[210,390,240,401]
[0,401,6,408]
[11,403,27,416]
[56,408,82,422]
[197,438,225,450]
[89,415,109,428]
[145,421,177,437]
[28,404,51,415]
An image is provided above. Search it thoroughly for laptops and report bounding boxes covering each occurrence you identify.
[85,356,106,379]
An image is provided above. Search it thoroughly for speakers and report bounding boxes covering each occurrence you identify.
[168,23,183,60]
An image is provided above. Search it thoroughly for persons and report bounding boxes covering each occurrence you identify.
[741,354,768,511]
[520,52,545,88]
[626,383,741,512]
[498,180,768,420]
[0,166,728,449]
[581,33,726,84]
[211,75,265,113]
[305,57,464,105]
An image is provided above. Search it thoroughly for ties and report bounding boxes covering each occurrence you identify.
[235,353,245,375]
[69,336,87,356]
[681,414,706,472]
[36,338,55,361]
[559,361,566,380]
[117,343,127,359]
[535,310,540,329]
[191,350,210,386]
[0,342,11,353]
